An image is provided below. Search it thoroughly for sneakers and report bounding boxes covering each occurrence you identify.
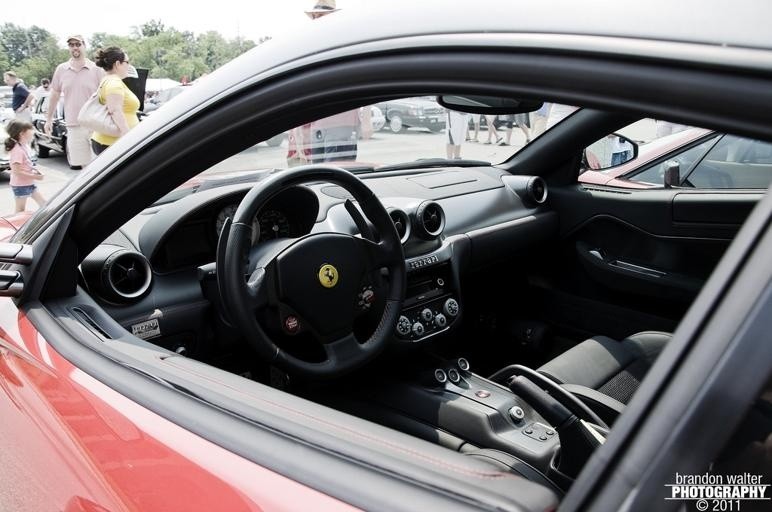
[466,134,511,146]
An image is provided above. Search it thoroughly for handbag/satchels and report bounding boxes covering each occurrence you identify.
[75,75,130,138]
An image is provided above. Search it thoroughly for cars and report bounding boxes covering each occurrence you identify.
[0,1,772,512]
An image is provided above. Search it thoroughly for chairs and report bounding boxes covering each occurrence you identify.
[463,448,565,502]
[538,330,680,401]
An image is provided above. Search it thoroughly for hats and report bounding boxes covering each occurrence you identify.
[66,34,86,46]
[304,0,343,15]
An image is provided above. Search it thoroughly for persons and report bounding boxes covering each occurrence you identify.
[3,118,47,213]
[43,34,107,169]
[2,71,37,123]
[444,100,694,170]
[35,76,56,113]
[90,45,141,156]
[304,0,374,164]
